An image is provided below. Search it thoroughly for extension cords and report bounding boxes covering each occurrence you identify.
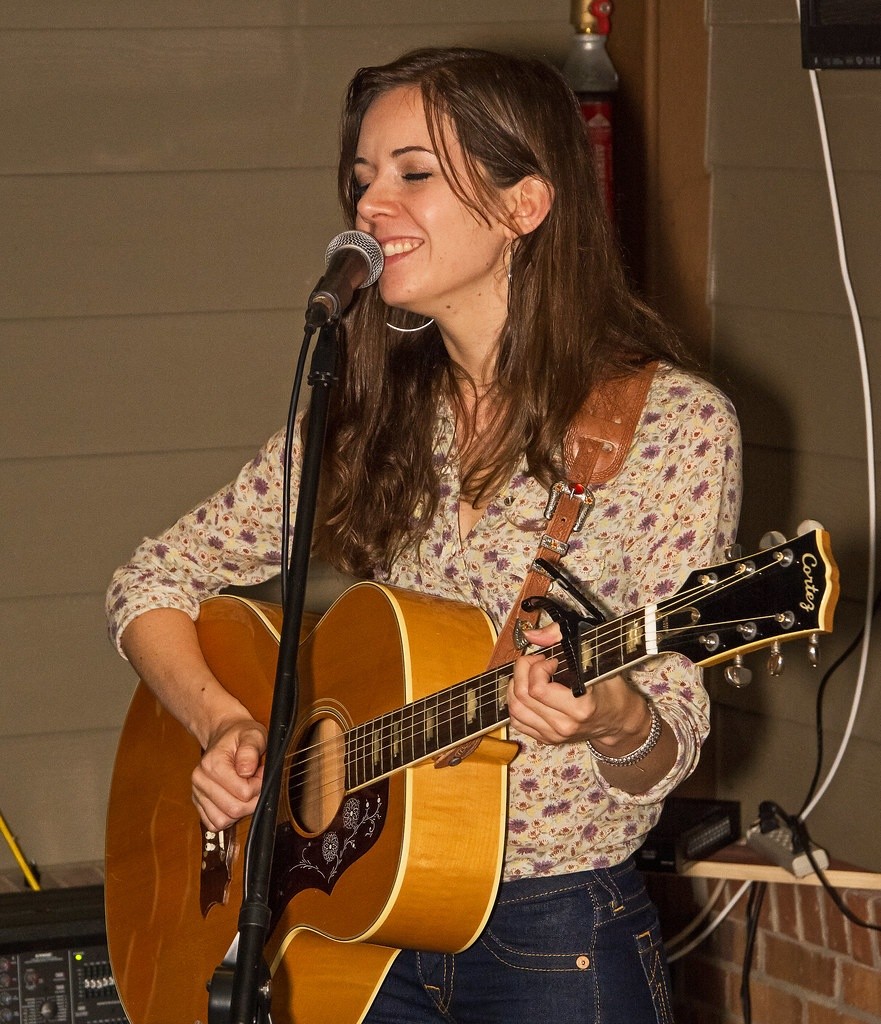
[746,824,830,877]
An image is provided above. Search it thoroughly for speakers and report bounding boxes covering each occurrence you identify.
[800,0,881,70]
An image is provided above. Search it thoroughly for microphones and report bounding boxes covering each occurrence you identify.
[304,230,384,334]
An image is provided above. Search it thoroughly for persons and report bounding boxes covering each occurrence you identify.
[104,46,746,1024]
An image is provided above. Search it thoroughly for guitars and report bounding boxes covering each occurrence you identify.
[101,518,839,1023]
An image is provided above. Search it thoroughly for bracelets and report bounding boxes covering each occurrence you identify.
[585,699,663,768]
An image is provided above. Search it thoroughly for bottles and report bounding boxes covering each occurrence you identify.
[552,1,624,238]
